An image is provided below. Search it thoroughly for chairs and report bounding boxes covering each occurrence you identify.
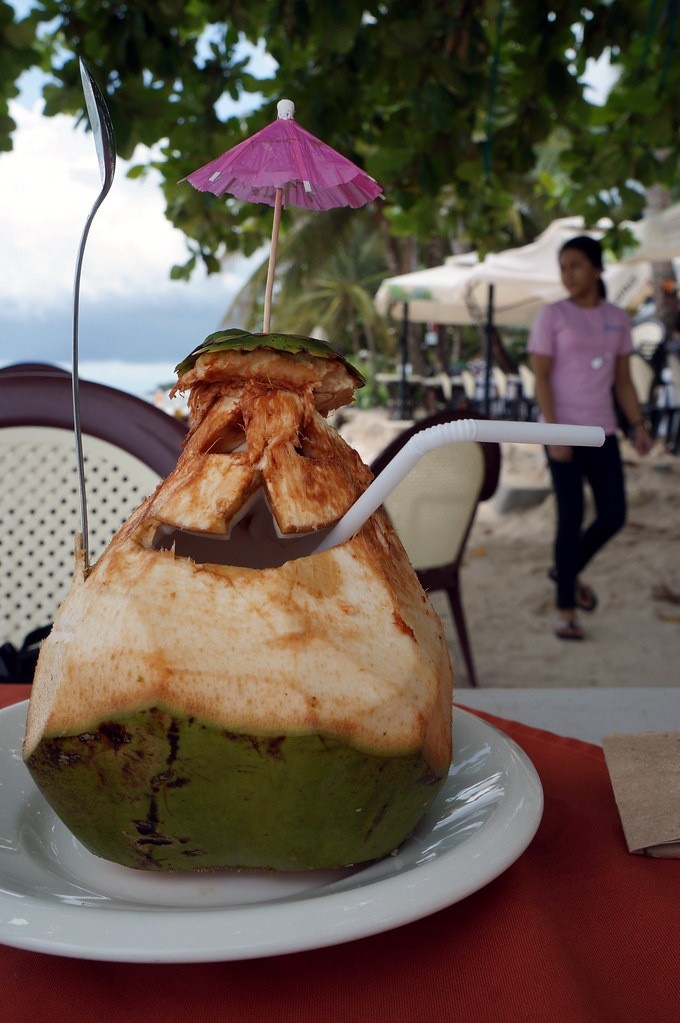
[491,365,523,420]
[627,349,667,442]
[367,407,504,686]
[517,362,539,423]
[439,371,466,408]
[460,369,488,412]
[0,358,188,654]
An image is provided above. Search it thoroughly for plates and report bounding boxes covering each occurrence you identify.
[0,699,543,964]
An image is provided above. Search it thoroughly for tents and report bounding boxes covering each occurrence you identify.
[373,215,657,416]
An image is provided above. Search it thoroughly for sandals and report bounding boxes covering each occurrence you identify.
[549,566,598,609]
[555,618,583,638]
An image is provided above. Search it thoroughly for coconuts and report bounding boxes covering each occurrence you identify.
[18,330,455,875]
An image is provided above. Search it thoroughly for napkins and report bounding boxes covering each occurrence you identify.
[599,725,680,861]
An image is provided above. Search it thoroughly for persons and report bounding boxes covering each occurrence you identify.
[529,236,653,642]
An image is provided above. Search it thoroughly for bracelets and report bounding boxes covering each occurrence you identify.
[633,419,645,427]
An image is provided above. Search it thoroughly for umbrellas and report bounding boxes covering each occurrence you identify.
[177,99,385,332]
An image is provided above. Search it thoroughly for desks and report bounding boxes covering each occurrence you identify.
[0,681,680,1023]
